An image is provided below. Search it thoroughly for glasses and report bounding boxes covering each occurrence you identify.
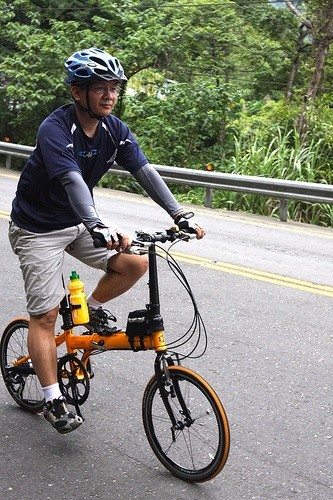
[83,84,121,95]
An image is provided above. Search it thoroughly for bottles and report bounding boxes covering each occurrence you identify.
[69,271,89,324]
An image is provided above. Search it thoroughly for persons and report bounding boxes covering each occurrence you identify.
[9,46,206,434]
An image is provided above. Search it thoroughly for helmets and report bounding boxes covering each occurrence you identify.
[63,48,128,85]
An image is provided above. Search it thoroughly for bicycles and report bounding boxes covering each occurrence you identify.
[0,227,232,484]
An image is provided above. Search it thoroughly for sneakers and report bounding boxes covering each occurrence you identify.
[82,305,114,331]
[42,396,83,434]
